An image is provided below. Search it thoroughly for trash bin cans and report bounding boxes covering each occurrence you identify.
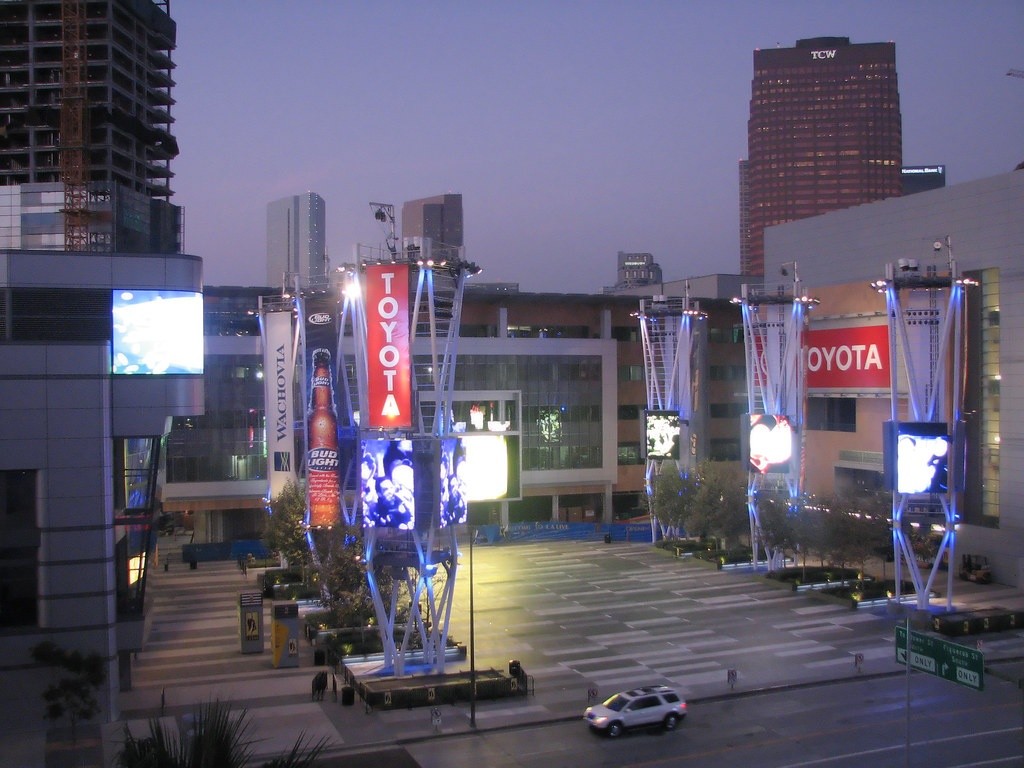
[605,533,612,544]
[342,687,355,705]
[314,649,326,666]
[510,660,521,676]
[263,584,273,598]
[190,556,197,569]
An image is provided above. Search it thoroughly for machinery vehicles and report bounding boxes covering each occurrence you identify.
[959,553,992,584]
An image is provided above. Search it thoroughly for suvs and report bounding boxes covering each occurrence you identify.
[582,685,688,739]
